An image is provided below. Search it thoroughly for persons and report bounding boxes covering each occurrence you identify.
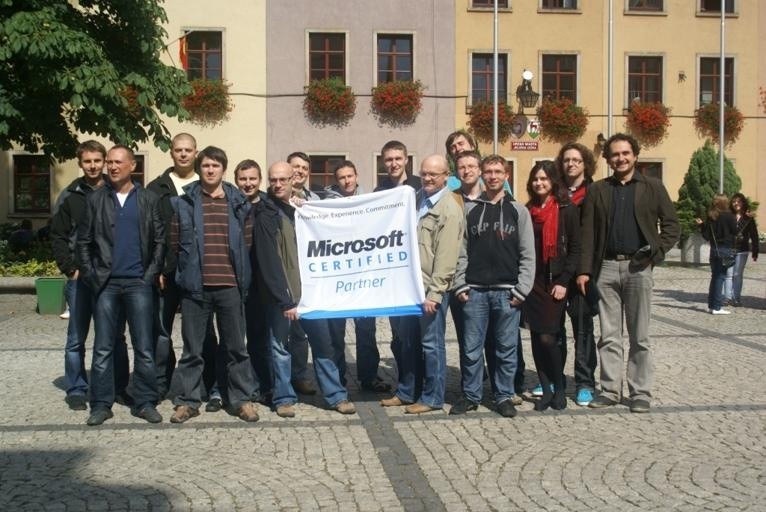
[722,193,759,305]
[50,130,683,429]
[694,194,738,314]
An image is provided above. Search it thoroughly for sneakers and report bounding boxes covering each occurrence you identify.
[706,298,740,314]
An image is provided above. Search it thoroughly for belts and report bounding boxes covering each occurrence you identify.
[614,253,633,260]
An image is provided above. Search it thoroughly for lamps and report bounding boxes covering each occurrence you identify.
[597,133,607,151]
[518,69,539,108]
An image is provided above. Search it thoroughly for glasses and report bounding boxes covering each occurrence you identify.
[270,174,295,185]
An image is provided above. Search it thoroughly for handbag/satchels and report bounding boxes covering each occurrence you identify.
[719,256,735,267]
[733,233,744,251]
[549,257,568,277]
[586,281,600,306]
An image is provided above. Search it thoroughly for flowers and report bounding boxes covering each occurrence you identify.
[182,80,235,128]
[463,100,515,147]
[694,103,746,150]
[118,85,143,120]
[368,81,430,130]
[537,92,588,142]
[302,76,357,130]
[625,100,672,149]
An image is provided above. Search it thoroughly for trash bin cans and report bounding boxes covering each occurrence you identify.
[36,277,65,315]
